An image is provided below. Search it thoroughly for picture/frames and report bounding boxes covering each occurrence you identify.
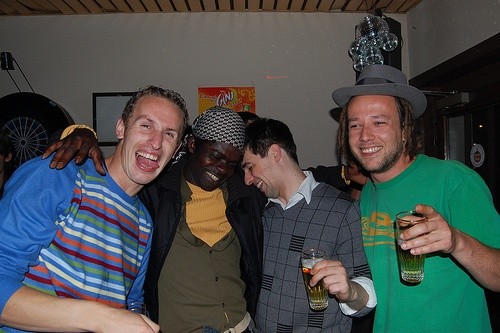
[92,92,139,146]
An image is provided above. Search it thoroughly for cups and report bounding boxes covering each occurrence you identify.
[301,249,328,311]
[120,302,146,317]
[395,210,428,283]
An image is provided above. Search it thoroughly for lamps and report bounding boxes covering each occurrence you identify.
[348,0,398,73]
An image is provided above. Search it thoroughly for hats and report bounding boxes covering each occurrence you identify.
[332,64,427,119]
[191,105,246,150]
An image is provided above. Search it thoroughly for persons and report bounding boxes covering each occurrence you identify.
[241,118,377,333]
[0,85,189,333]
[41,105,366,333]
[332,66,500,333]
